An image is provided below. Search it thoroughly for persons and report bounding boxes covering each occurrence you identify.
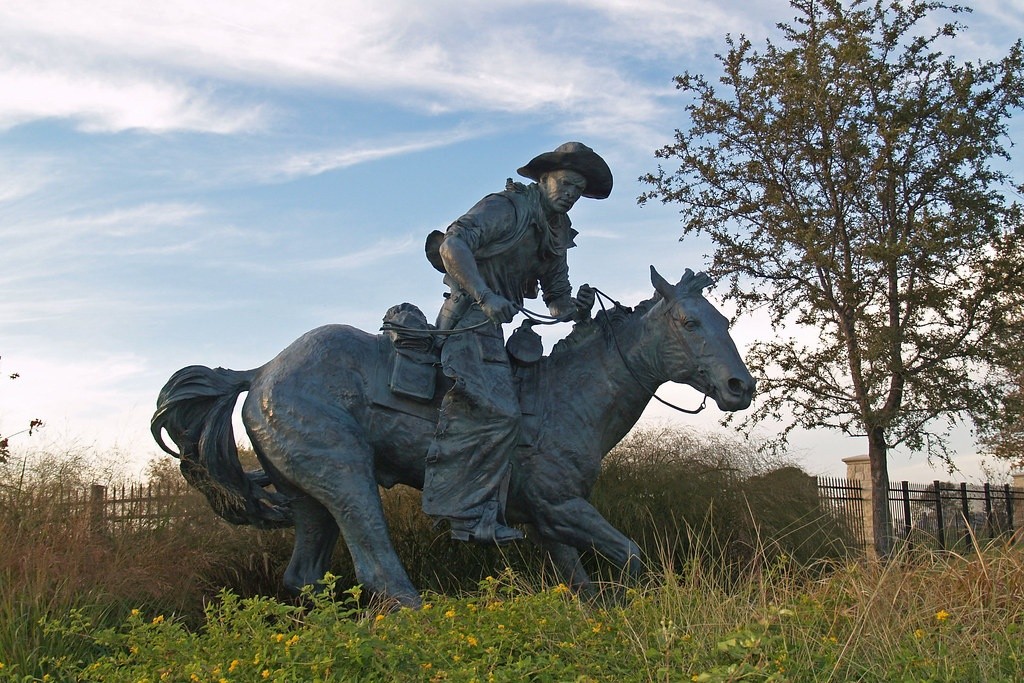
[424,141,613,544]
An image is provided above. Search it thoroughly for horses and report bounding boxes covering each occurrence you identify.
[150,265,759,614]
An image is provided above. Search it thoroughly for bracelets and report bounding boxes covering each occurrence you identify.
[477,288,492,305]
